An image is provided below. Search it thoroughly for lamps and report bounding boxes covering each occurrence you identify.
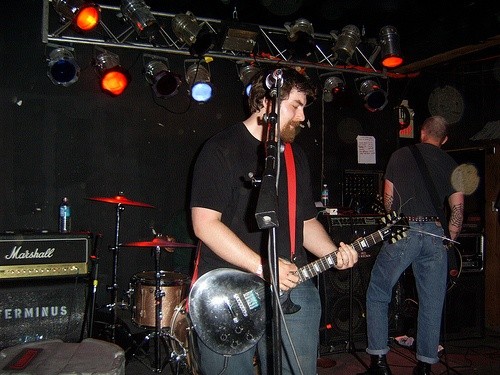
[53,0,102,32]
[376,26,404,68]
[93,46,127,96]
[44,42,81,88]
[220,19,261,53]
[142,52,180,99]
[172,11,213,54]
[320,70,347,98]
[236,61,262,96]
[183,57,212,104]
[353,74,390,111]
[284,18,314,49]
[330,25,365,61]
[120,0,159,39]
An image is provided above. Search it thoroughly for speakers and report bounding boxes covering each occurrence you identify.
[314,214,406,345]
[401,272,484,341]
[0,283,92,352]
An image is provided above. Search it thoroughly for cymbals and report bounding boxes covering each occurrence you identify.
[120,238,198,247]
[86,195,156,207]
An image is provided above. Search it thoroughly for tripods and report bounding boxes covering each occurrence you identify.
[124,248,181,375]
[97,202,152,358]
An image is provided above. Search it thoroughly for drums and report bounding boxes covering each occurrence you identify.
[131,270,187,332]
[169,296,191,374]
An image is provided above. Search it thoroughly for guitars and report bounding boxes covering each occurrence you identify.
[187,210,411,356]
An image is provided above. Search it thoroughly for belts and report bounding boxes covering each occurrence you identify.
[402,216,440,222]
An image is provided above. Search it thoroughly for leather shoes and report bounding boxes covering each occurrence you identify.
[354,354,394,375]
[413,361,432,375]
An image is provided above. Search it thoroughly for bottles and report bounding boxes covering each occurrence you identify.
[59,196,71,232]
[322,184,329,206]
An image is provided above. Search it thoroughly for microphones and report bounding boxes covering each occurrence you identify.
[266,68,284,90]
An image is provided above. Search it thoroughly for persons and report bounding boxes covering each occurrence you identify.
[189,63,359,375]
[363,115,463,375]
